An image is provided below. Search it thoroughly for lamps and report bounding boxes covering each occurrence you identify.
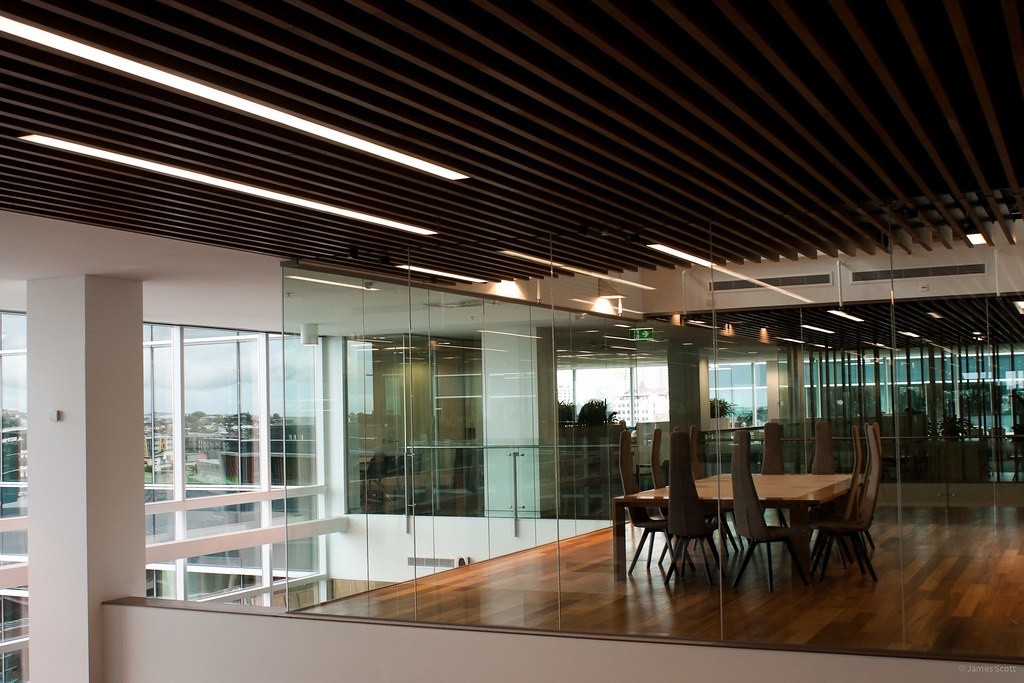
[599,279,628,299]
[966,224,987,245]
[300,324,318,345]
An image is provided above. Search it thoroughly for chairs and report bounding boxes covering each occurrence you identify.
[731,420,883,593]
[619,425,740,586]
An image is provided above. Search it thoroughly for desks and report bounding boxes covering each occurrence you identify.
[613,474,865,579]
